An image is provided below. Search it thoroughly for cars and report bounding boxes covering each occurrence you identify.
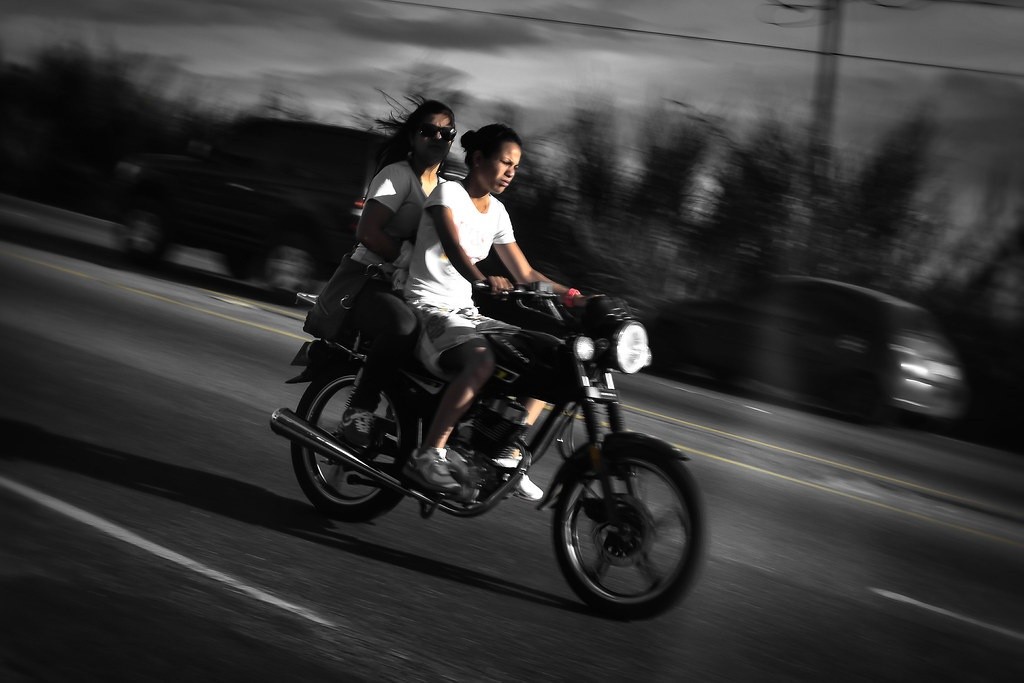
[659,269,978,438]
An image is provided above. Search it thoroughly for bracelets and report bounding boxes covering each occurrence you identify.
[565,288,581,309]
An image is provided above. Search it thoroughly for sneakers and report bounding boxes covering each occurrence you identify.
[336,407,377,448]
[404,446,461,492]
[495,459,544,500]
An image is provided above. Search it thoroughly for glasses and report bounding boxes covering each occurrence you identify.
[416,122,457,141]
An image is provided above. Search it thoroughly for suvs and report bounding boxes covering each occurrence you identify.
[119,114,474,308]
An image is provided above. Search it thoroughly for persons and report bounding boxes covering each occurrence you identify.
[339,98,456,459]
[402,121,591,501]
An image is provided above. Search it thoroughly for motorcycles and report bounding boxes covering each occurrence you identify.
[270,282,716,623]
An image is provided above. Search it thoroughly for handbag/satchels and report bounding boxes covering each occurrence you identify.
[303,255,381,352]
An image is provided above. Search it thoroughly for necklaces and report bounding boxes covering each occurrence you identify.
[479,203,487,214]
